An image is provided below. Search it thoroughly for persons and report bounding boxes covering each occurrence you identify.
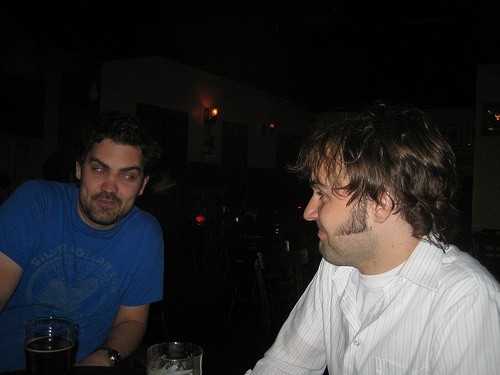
[246,98,500,375]
[0,119,164,372]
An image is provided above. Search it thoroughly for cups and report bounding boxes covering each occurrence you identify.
[146,342,203,375]
[23,317,78,374]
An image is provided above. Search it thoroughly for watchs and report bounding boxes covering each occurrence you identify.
[96,347,122,366]
